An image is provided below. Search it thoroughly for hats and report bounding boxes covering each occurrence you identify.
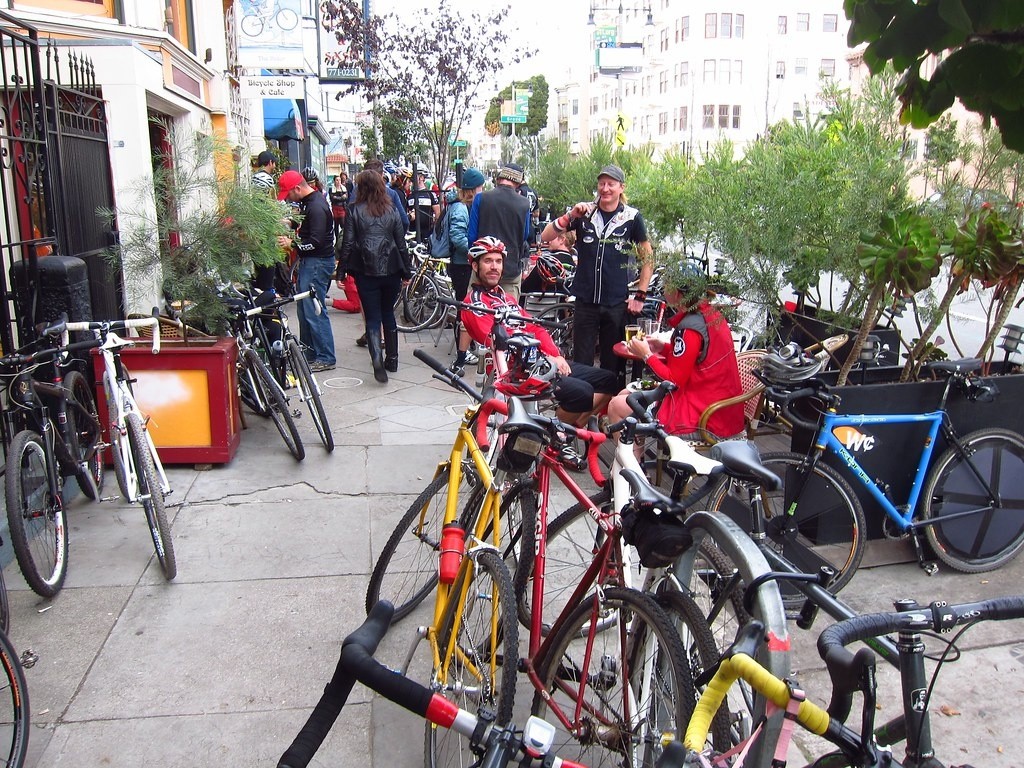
[462,167,486,190]
[277,170,303,200]
[258,151,279,165]
[597,164,626,183]
[498,162,524,185]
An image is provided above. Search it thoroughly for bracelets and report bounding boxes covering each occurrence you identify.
[634,290,646,303]
[551,211,572,232]
[642,352,653,364]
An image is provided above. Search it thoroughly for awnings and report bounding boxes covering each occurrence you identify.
[262,68,306,143]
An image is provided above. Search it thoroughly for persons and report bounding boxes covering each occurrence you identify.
[278,171,336,372]
[607,261,746,468]
[540,166,655,440]
[290,167,335,245]
[325,160,411,348]
[462,236,616,469]
[340,173,353,203]
[172,203,306,388]
[383,164,446,238]
[328,176,348,240]
[336,169,407,383]
[430,163,577,378]
[251,152,279,293]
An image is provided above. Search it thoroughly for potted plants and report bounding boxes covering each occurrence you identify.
[756,68,933,368]
[89,128,307,465]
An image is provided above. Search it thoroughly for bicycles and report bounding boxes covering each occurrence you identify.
[700,358,1024,607]
[0,312,109,597]
[0,525,42,768]
[175,150,1024,768]
[42,307,178,581]
[240,0,299,37]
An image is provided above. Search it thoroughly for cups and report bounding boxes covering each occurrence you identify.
[625,324,641,344]
[637,317,661,340]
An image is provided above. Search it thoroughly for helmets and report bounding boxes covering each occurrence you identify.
[661,260,707,295]
[381,162,430,183]
[301,166,319,183]
[466,236,508,267]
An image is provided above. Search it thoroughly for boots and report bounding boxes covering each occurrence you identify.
[383,327,399,372]
[367,328,388,383]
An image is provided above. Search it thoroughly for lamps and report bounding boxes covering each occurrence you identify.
[715,258,729,274]
[204,48,212,63]
[997,324,1024,355]
[163,6,173,32]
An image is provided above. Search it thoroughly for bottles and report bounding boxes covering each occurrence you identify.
[271,340,283,365]
[440,519,465,584]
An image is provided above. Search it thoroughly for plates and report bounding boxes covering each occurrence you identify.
[626,380,661,393]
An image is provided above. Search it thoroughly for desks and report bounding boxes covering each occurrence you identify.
[614,334,668,384]
[709,293,741,324]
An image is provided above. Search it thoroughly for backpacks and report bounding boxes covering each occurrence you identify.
[426,198,471,259]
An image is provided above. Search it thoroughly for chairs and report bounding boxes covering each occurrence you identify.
[525,247,772,518]
[126,312,249,430]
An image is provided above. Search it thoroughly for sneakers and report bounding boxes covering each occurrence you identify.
[598,415,614,438]
[552,433,588,470]
[455,348,479,365]
[307,359,316,364]
[309,361,337,372]
[449,360,465,377]
